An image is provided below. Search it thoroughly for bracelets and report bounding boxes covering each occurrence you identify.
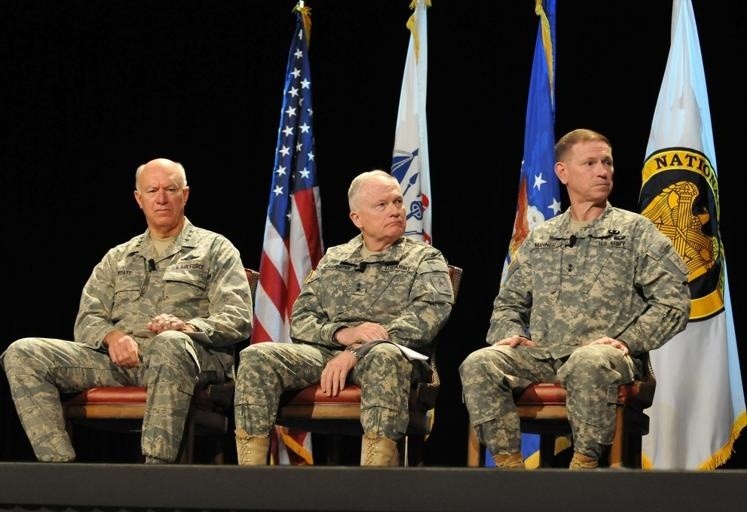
[346,345,362,362]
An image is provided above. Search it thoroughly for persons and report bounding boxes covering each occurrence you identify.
[2,158,255,466]
[458,127,693,470]
[231,169,456,468]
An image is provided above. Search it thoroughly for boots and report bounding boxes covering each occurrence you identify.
[359,431,400,468]
[234,428,273,466]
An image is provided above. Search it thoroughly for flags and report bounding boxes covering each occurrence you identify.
[392,0,437,445]
[638,1,747,472]
[483,4,565,471]
[249,0,326,467]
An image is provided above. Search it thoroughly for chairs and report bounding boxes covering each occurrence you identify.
[274,264,465,466]
[58,264,262,468]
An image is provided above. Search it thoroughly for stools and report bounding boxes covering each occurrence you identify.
[464,379,655,468]
